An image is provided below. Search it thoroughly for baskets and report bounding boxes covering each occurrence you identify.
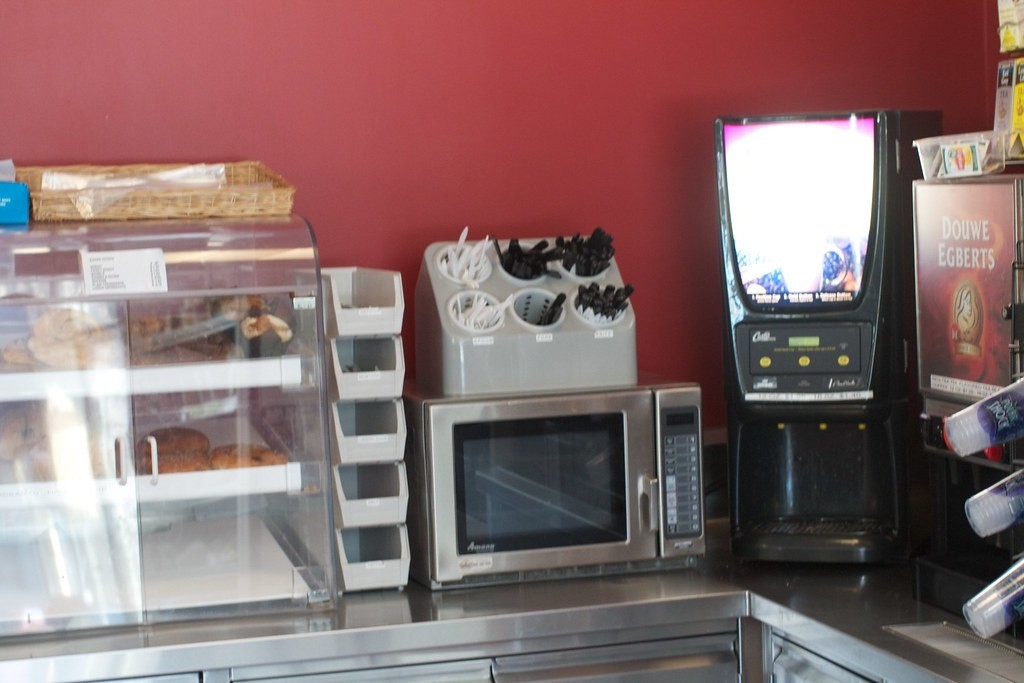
[13,161,295,221]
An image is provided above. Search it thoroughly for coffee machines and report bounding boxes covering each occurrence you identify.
[714,107,945,565]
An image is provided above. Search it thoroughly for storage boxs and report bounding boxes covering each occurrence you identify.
[295,461,409,528]
[286,334,406,400]
[290,398,406,463]
[294,264,407,336]
[913,129,1014,181]
[295,524,411,593]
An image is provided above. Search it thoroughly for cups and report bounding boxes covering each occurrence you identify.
[962,557,1024,639]
[965,467,1024,538]
[944,376,1024,457]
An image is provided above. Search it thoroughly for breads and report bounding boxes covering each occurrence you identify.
[0,409,288,473]
[0,287,291,371]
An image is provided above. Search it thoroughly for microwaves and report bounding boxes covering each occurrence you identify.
[402,383,707,591]
